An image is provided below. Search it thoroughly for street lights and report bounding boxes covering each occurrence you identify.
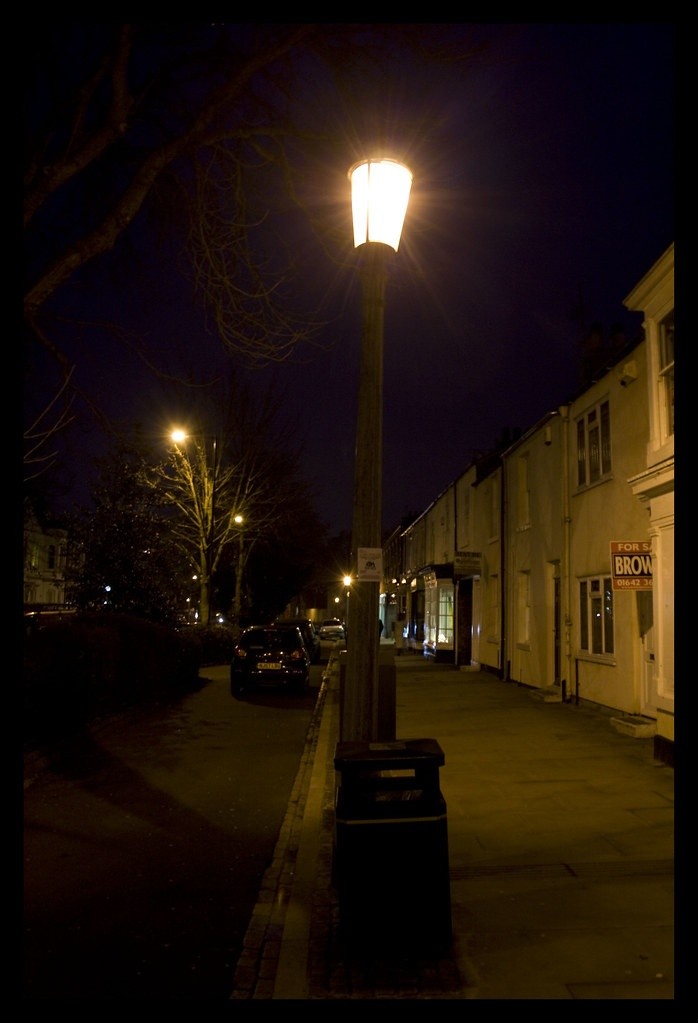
[337,137,419,747]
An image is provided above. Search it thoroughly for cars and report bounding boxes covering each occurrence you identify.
[318,619,346,640]
[227,624,311,701]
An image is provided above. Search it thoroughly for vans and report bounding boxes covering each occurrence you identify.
[271,618,320,667]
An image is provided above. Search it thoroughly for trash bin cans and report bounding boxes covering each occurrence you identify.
[335,739,454,957]
